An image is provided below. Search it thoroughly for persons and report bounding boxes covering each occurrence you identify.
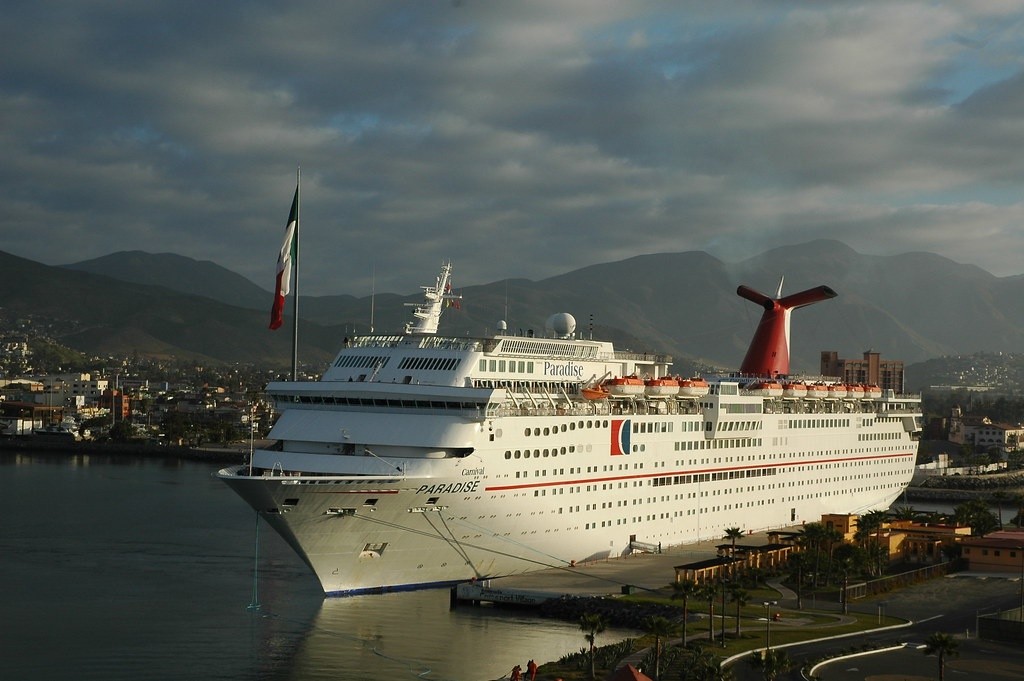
[658,541,661,553]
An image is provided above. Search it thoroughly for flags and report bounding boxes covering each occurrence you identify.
[269,187,298,330]
[446,274,462,310]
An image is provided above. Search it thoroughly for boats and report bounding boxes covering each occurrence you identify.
[762,380,882,398]
[582,375,710,399]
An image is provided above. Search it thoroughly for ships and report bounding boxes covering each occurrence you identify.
[218,262,923,595]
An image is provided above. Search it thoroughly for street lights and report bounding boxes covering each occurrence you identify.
[764,600,778,649]
